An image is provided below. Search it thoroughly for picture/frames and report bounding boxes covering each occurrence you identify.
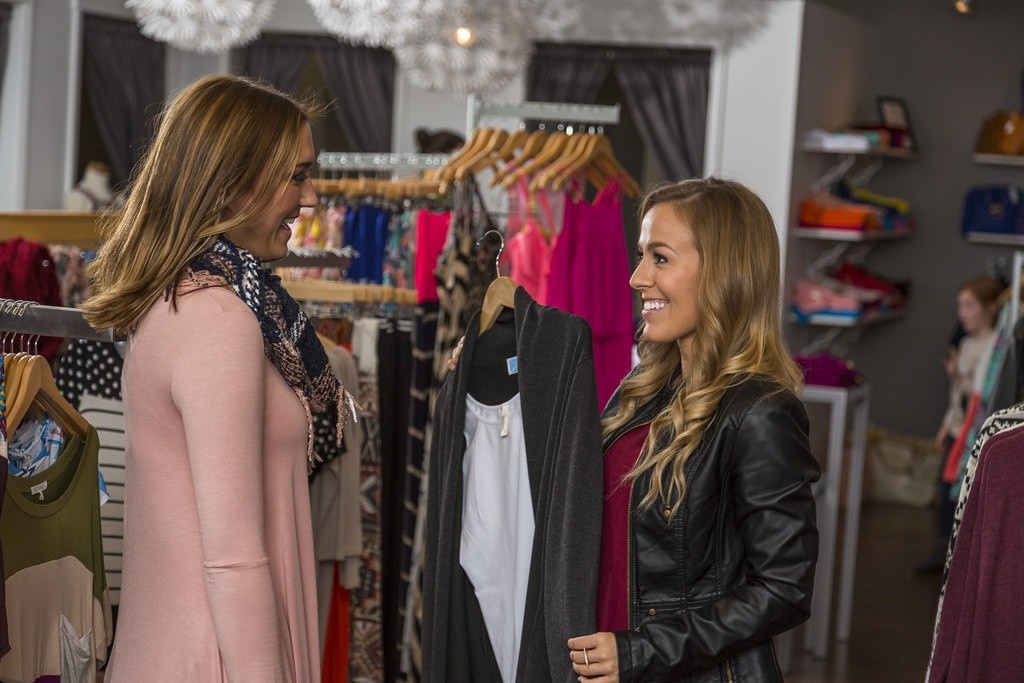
[877,96,913,134]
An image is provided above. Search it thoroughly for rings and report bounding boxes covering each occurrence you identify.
[583,648,590,669]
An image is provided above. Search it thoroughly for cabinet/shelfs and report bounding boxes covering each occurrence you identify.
[787,142,917,326]
[967,152,1024,245]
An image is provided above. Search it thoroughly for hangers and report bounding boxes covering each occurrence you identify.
[275,244,415,322]
[310,98,641,210]
[0,298,89,444]
[476,229,517,338]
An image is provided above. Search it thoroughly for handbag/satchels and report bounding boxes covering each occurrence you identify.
[961,182,1024,236]
[976,110,1024,155]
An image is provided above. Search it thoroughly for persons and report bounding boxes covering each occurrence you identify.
[447,175,823,683]
[419,130,464,154]
[81,72,349,682]
[916,273,1006,574]
[63,163,115,213]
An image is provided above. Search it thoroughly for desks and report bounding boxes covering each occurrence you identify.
[773,381,871,674]
